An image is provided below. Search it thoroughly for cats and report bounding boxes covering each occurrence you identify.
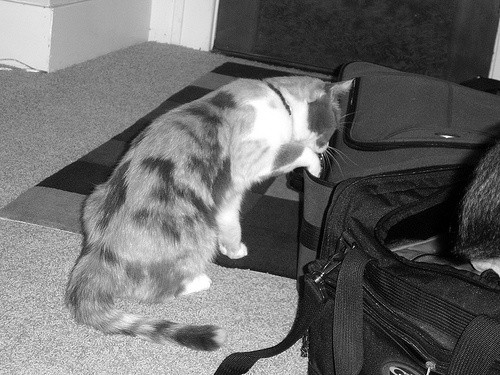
[64,75,373,352]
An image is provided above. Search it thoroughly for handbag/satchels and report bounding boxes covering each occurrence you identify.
[296,61,499,289]
[219,163,499,375]
